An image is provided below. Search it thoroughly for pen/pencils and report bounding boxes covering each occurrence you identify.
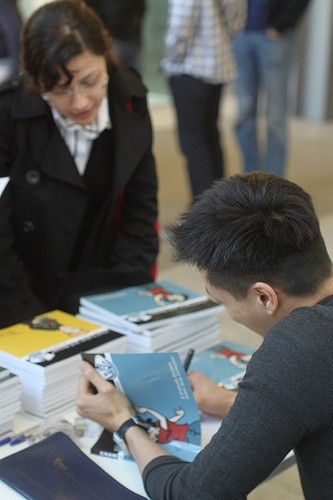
[0,425,39,445]
[184,350,194,372]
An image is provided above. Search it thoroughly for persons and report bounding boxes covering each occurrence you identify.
[164,0,247,199]
[76,169,333,500]
[0,0,160,328]
[231,0,314,177]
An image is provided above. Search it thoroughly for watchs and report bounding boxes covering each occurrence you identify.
[113,415,153,441]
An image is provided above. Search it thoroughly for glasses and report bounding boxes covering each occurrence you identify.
[41,72,110,101]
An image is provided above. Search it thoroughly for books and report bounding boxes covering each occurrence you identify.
[0,278,226,441]
[180,339,260,392]
[0,432,149,500]
[80,351,226,464]
